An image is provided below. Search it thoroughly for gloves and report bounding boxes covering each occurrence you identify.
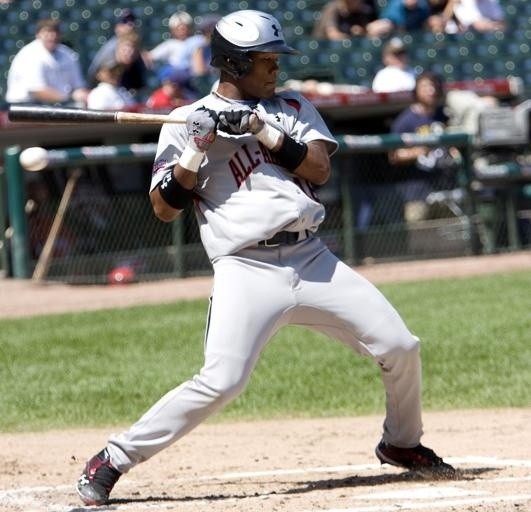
[186,105,265,152]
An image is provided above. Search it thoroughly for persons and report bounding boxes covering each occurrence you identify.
[74,9,459,508]
[1,0,531,262]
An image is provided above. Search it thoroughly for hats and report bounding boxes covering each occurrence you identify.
[382,39,407,54]
[113,8,137,22]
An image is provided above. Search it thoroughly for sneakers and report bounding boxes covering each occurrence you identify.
[376,436,455,480]
[77,446,122,506]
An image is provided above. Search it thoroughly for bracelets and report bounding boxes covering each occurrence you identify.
[274,132,309,173]
[178,145,205,175]
[252,122,282,150]
[158,169,198,209]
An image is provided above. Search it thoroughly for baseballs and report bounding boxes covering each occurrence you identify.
[20,147,49,171]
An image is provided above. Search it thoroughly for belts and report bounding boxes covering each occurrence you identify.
[259,228,309,247]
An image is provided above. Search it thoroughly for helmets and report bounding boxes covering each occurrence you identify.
[210,9,300,81]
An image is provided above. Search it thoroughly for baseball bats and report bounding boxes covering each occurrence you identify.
[8,103,258,131]
